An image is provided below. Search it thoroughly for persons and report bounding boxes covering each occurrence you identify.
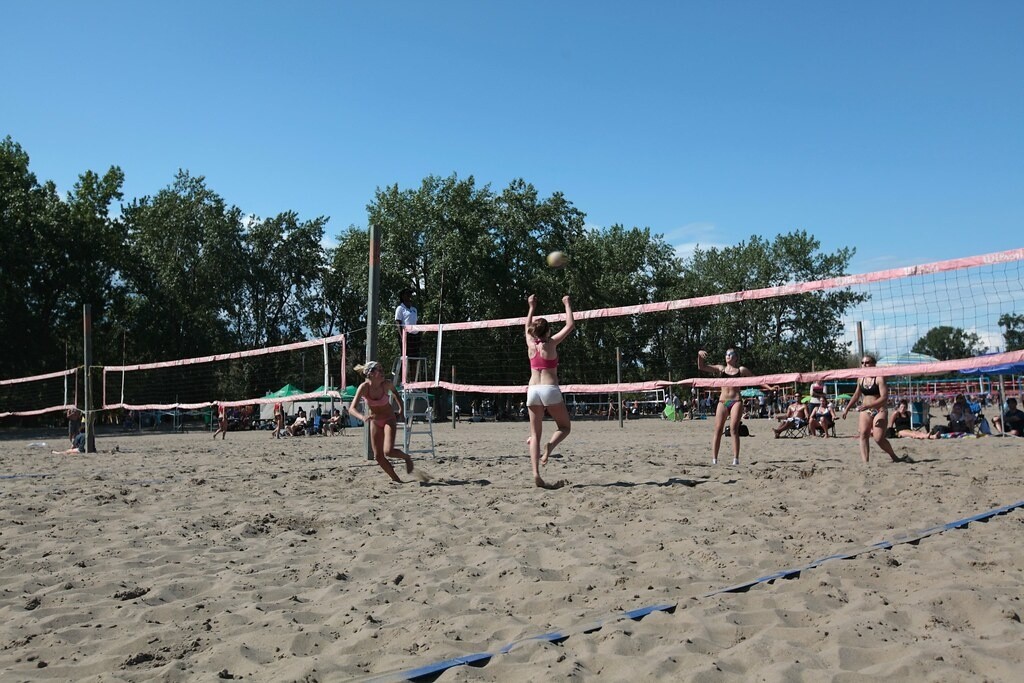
[50,427,86,454]
[842,355,901,463]
[805,380,827,437]
[213,404,436,440]
[698,345,780,465]
[154,413,161,431]
[524,294,575,487]
[455,391,721,424]
[743,389,1024,439]
[66,409,83,443]
[348,361,415,481]
[122,416,134,433]
[271,403,282,439]
[395,288,422,382]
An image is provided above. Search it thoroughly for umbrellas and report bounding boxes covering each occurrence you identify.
[876,351,941,402]
[740,388,764,413]
[258,383,434,427]
[957,353,1024,402]
[664,404,684,421]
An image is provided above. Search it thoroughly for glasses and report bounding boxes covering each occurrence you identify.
[861,362,874,365]
[726,352,735,355]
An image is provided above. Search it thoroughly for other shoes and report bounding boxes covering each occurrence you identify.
[402,389,413,393]
[411,389,423,393]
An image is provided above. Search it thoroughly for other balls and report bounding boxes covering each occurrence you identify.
[548,251,563,267]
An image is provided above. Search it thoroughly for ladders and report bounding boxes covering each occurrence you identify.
[388,356,436,460]
